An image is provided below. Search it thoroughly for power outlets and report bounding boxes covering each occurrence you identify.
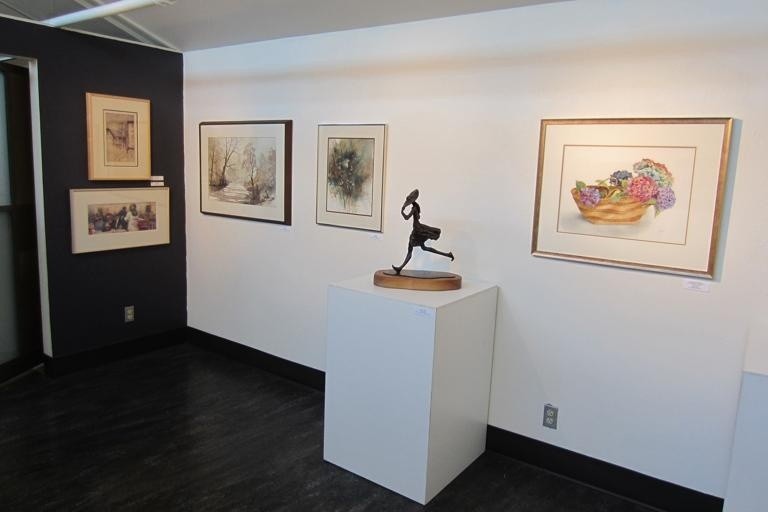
[542,404,558,431]
[125,305,134,323]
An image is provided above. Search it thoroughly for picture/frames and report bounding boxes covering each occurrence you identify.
[86,91,151,181]
[69,186,171,254]
[199,120,292,226]
[316,124,385,233]
[531,117,733,280]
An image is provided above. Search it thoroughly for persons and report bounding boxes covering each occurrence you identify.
[88,204,156,234]
[391,190,454,275]
